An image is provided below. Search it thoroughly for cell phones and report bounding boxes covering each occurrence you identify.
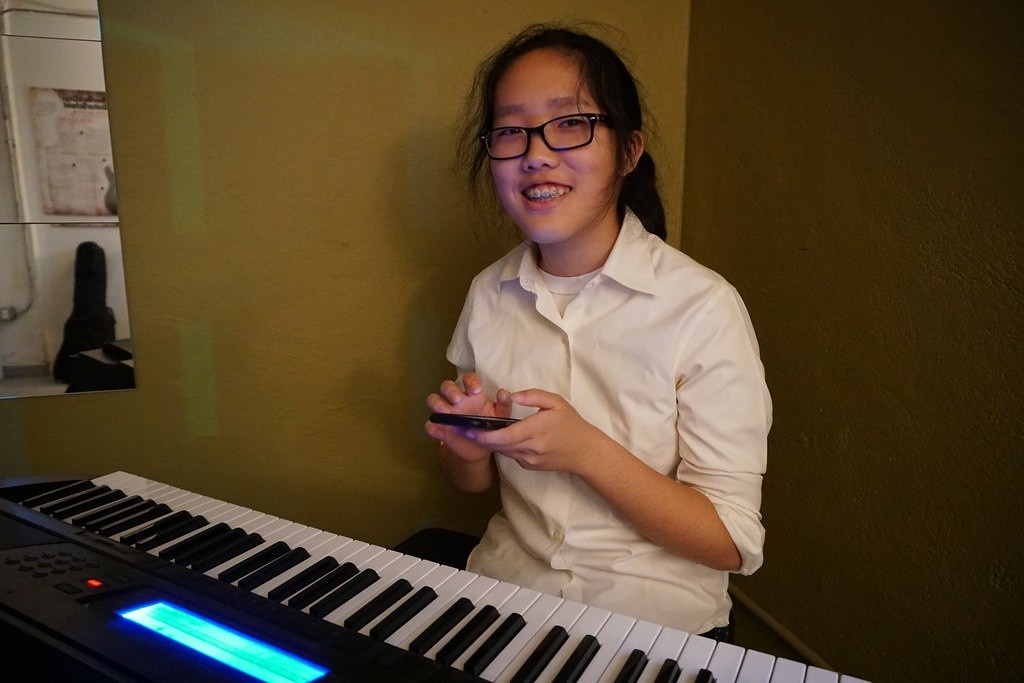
[429,413,521,431]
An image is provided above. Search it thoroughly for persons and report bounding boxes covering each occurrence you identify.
[405,19,776,637]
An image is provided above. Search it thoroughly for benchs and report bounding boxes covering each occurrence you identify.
[390,528,481,571]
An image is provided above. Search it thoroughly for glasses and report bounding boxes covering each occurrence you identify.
[479,112,610,160]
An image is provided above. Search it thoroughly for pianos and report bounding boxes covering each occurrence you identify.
[0,467,877,683]
